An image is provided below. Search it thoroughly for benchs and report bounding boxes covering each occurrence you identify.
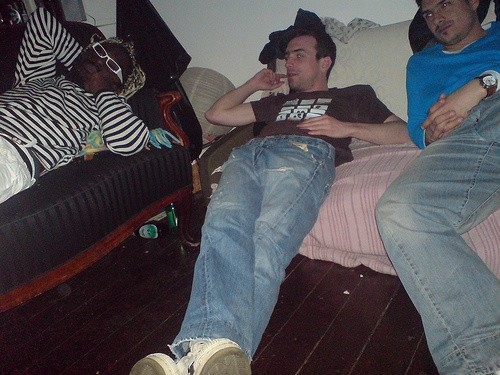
[0,21,195,316]
[198,0,500,280]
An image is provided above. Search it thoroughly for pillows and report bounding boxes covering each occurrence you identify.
[179,66,239,143]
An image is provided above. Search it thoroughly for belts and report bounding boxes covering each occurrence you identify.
[0,132,38,179]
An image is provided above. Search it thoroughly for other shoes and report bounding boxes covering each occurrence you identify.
[130,353,195,375]
[190,338,251,375]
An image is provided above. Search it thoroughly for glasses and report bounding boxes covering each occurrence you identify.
[91,42,123,84]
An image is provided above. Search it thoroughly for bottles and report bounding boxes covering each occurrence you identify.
[131,220,165,238]
[7,4,22,25]
[165,202,181,233]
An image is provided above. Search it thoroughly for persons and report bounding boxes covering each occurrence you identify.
[129,24,412,375]
[372,0,500,374]
[0,7,150,202]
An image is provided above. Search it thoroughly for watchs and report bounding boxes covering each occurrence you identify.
[474,72,497,98]
